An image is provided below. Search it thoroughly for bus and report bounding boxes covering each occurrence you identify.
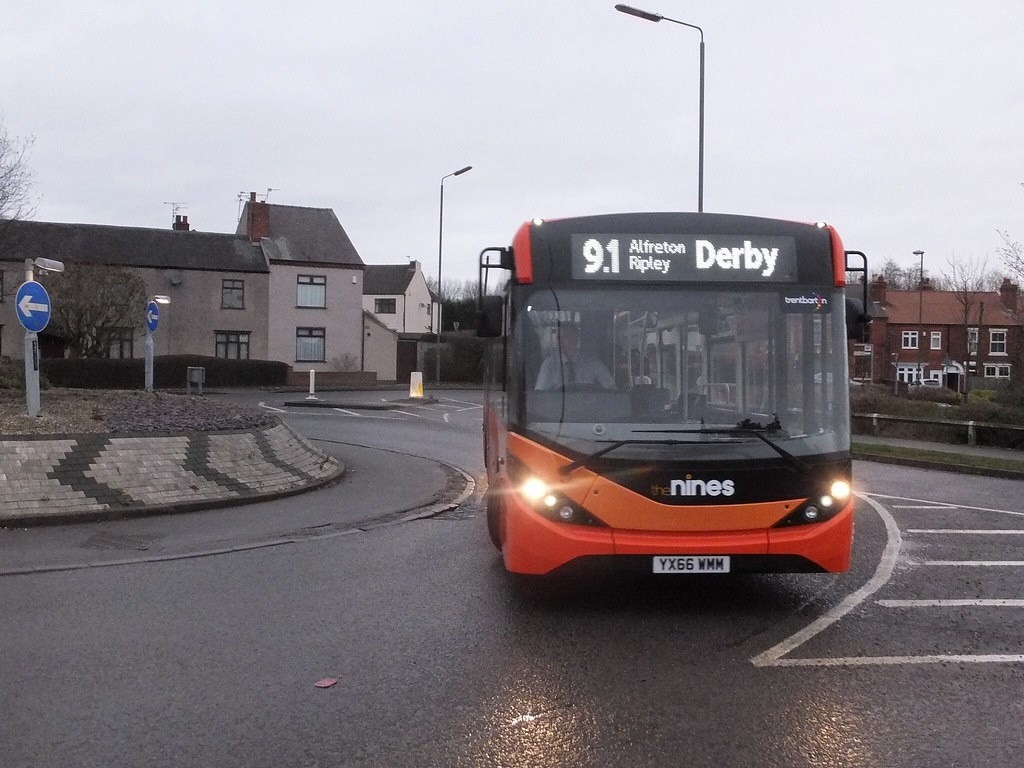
[476,213,871,591]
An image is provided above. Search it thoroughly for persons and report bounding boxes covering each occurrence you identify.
[535,321,620,394]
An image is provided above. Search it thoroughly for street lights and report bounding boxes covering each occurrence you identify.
[615,3,706,214]
[144,293,171,390]
[912,250,926,385]
[21,256,67,414]
[434,166,474,385]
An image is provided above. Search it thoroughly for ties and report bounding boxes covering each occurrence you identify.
[567,361,575,384]
